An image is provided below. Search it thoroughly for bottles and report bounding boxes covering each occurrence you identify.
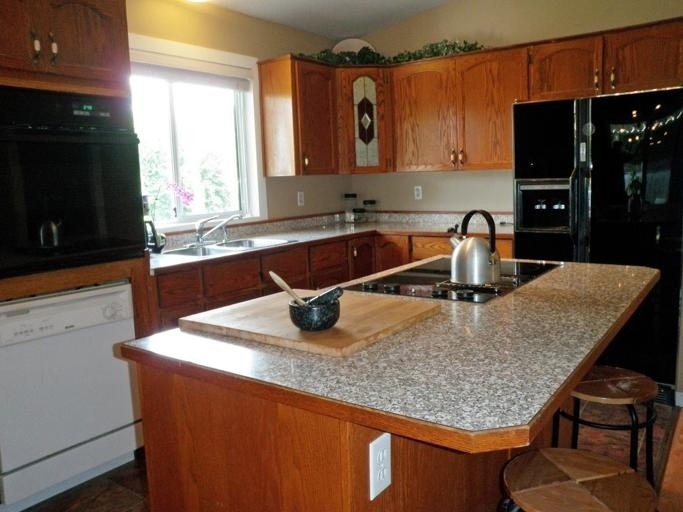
[342,191,376,225]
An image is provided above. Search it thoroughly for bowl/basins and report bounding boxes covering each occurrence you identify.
[289,295,339,331]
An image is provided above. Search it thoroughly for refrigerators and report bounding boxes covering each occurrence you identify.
[511,85,682,411]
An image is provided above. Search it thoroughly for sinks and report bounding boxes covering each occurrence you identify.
[214,237,296,250]
[161,244,240,259]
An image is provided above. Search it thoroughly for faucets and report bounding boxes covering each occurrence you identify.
[194,212,243,245]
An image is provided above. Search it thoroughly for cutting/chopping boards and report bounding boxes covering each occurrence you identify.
[178,286,442,357]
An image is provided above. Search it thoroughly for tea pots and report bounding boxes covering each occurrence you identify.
[444,210,502,284]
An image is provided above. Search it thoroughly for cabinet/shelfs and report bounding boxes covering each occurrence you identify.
[337,64,396,176]
[0,0,133,99]
[374,233,514,277]
[528,15,683,104]
[151,233,377,334]
[395,44,529,175]
[257,52,337,180]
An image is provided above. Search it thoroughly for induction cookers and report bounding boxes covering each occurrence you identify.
[339,259,559,302]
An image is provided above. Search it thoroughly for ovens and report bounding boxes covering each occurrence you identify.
[1,84,145,284]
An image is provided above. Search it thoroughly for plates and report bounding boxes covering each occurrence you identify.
[330,38,376,55]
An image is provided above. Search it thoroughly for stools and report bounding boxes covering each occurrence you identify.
[551,364,666,487]
[497,446,658,510]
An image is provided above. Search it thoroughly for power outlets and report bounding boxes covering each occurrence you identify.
[367,433,392,502]
[414,185,422,200]
[297,191,304,206]
